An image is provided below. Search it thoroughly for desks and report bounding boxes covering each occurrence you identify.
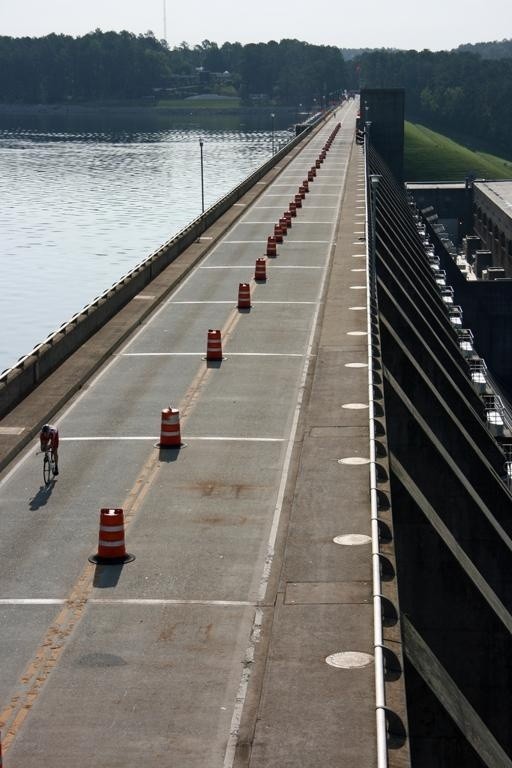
[297,87,349,129]
[198,134,207,215]
[270,111,276,155]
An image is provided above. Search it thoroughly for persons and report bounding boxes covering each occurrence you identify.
[40,423,60,476]
